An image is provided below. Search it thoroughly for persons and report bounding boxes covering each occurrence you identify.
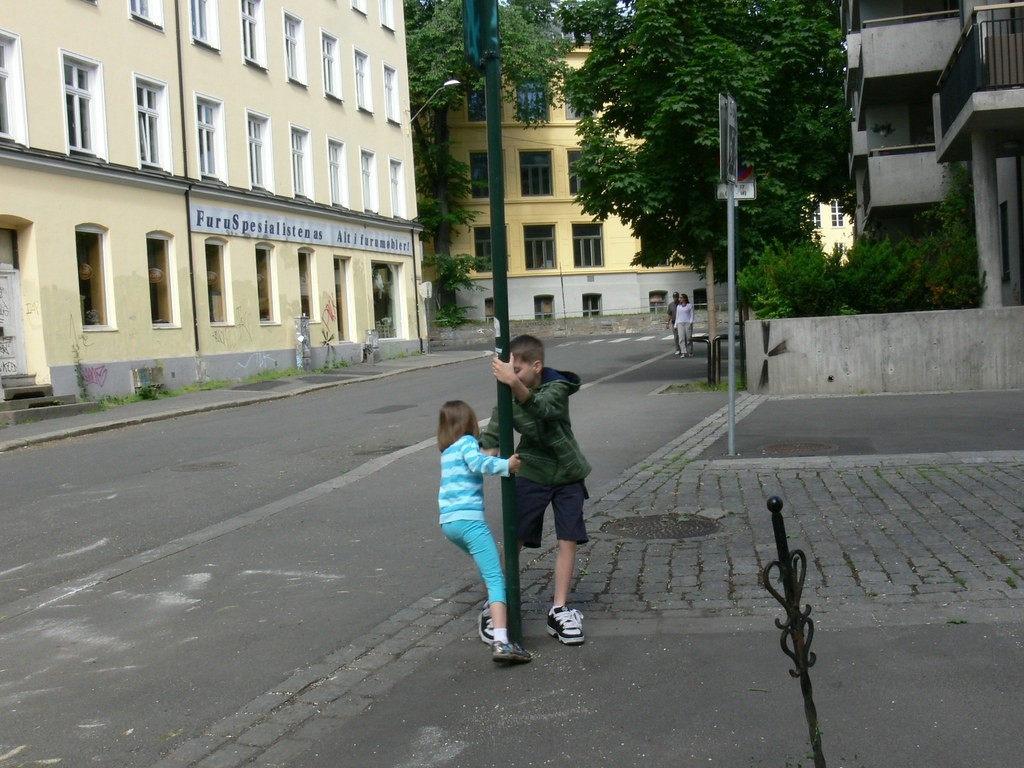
[479,335,592,647]
[438,400,531,662]
[666,292,694,358]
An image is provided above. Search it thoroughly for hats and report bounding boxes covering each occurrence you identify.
[672,292,679,297]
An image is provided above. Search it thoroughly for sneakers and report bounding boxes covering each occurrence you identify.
[478,599,494,646]
[491,641,531,662]
[546,604,585,645]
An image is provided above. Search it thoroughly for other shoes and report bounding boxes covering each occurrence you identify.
[681,354,685,358]
[689,353,693,357]
[675,350,680,355]
